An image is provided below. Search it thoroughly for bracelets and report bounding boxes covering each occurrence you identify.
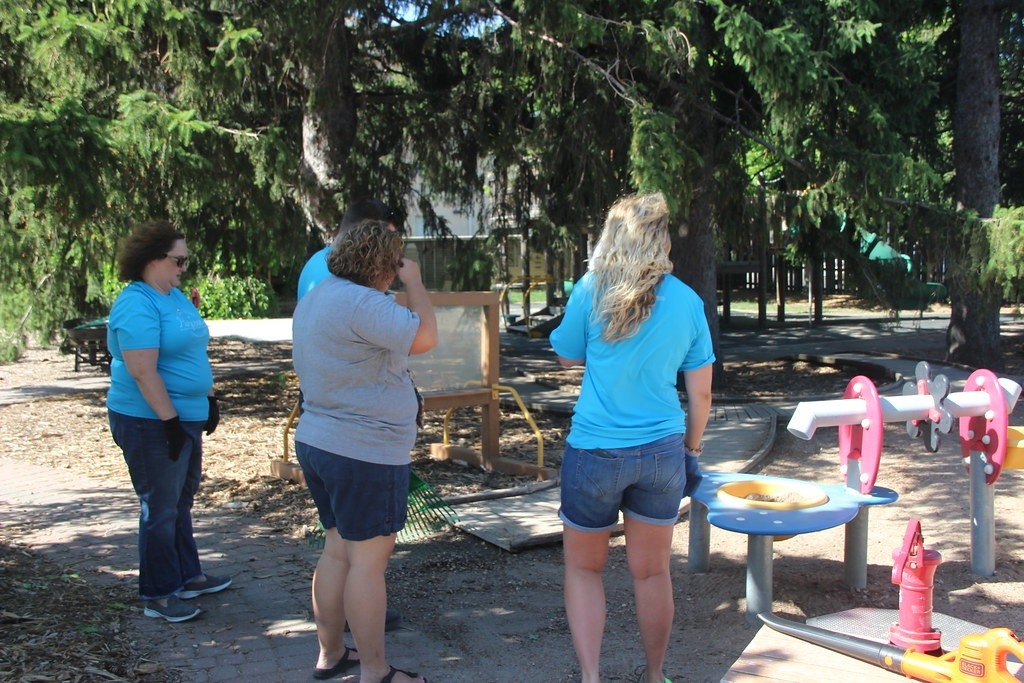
[683,441,703,452]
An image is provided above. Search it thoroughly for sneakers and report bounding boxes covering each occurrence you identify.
[144,596,201,622]
[175,573,232,599]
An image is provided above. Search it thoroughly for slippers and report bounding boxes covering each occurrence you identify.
[312,646,361,679]
[379,665,427,683]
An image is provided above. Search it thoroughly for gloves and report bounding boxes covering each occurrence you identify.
[681,444,703,497]
[162,416,186,461]
[203,394,220,436]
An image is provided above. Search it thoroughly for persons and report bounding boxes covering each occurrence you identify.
[295,217,437,683]
[292,199,404,635]
[548,193,716,683]
[106,219,234,625]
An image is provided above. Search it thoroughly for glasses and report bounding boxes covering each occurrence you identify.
[158,251,190,269]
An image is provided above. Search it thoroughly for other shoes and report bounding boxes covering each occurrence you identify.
[344,608,402,632]
[639,668,672,683]
